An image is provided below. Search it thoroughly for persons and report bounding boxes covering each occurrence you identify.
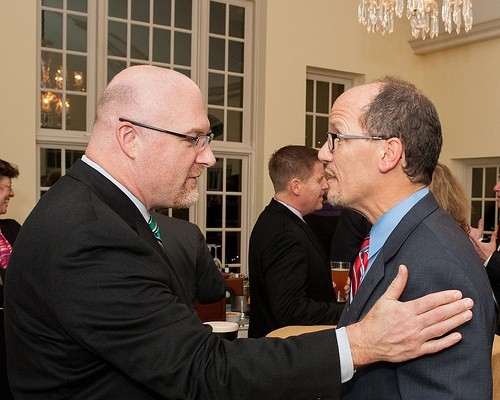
[316,73,498,400]
[149,209,227,317]
[5,66,473,400]
[246,144,350,340]
[303,159,499,339]
[1,157,23,399]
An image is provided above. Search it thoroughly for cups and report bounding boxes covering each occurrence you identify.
[331,261,350,302]
[203,321,238,342]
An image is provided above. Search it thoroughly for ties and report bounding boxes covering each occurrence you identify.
[148,217,161,247]
[348,235,369,308]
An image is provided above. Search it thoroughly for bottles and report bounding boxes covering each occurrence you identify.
[225,262,249,286]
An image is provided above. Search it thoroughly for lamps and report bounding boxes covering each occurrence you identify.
[352,1,478,41]
[38,41,85,125]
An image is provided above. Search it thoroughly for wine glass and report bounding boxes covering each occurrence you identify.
[210,244,221,272]
[207,243,213,253]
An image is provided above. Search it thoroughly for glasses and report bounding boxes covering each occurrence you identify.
[326,132,383,152]
[118,118,214,151]
